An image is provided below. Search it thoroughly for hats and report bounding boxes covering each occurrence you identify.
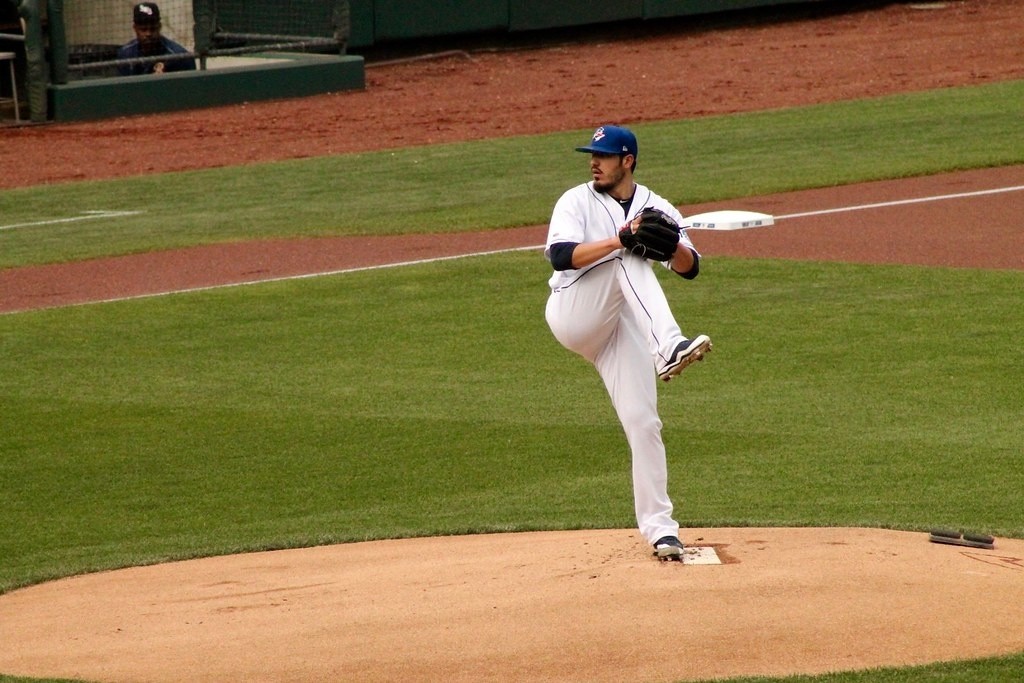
[575,125,638,156]
[133,3,160,26]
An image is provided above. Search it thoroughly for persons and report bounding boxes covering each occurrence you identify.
[544,125,713,561]
[111,2,196,77]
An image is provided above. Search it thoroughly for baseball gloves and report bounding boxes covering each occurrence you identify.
[619,205,681,261]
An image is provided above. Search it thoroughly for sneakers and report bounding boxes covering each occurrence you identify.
[653,535,684,562]
[658,335,713,382]
